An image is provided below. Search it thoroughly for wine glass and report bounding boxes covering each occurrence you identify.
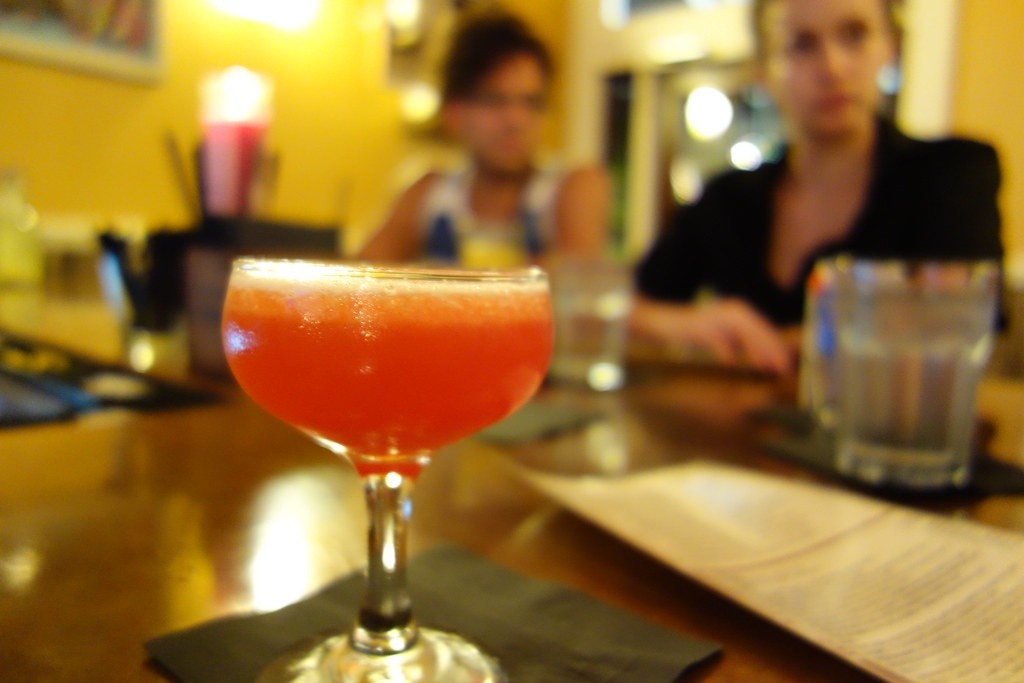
[221,259,554,683]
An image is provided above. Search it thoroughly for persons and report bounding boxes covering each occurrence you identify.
[358,12,608,281]
[622,0,1009,375]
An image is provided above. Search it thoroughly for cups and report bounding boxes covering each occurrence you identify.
[819,260,996,485]
[548,269,630,389]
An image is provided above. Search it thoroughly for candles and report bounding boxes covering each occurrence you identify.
[197,67,272,215]
